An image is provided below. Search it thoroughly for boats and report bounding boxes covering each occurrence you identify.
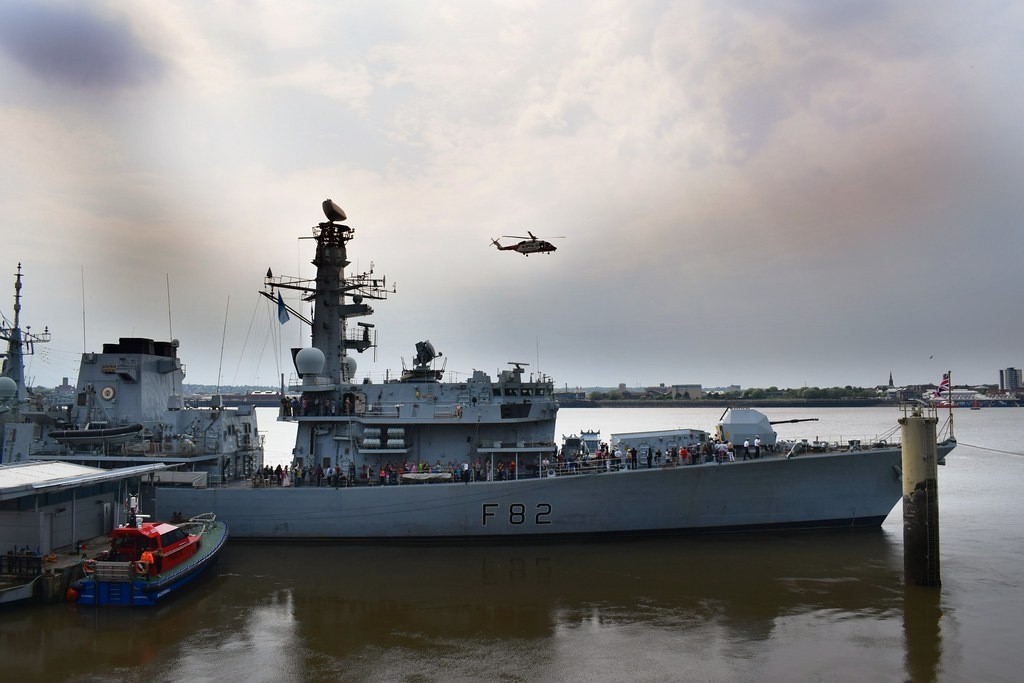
[0,545,47,605]
[69,511,230,608]
[47,423,144,448]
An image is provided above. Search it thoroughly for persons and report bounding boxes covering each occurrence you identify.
[286,396,354,416]
[264,435,761,488]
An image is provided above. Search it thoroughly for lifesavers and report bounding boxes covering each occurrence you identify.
[135,560,148,573]
[84,558,97,572]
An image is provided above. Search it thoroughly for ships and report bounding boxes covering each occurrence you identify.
[0,198,958,548]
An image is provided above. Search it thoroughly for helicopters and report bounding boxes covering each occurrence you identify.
[489,230,566,258]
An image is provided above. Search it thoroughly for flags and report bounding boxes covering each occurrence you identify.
[278,291,290,325]
